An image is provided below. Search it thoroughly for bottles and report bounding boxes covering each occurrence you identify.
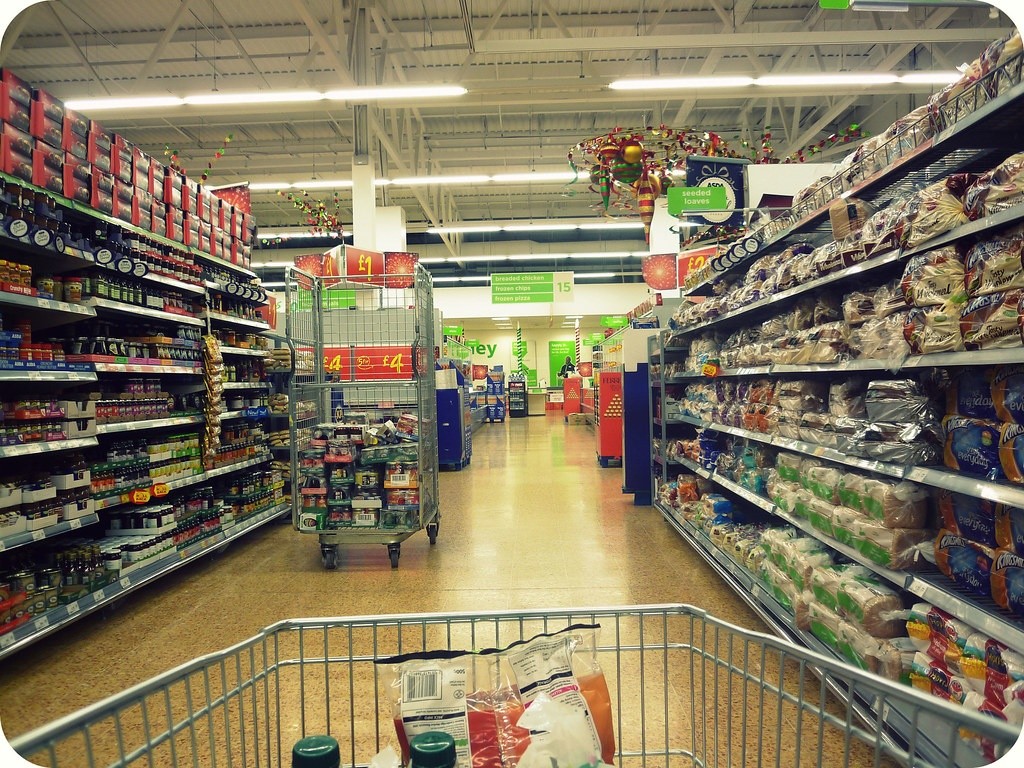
[0,177,287,633]
[511,402,524,407]
[299,422,419,529]
[291,735,342,768]
[408,731,460,768]
[510,392,524,398]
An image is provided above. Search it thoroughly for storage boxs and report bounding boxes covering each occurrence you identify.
[568,413,586,424]
[476,372,507,423]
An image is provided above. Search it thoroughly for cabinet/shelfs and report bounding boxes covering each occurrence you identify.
[286,263,441,568]
[0,169,294,661]
[508,382,525,416]
[648,80,1024,768]
[592,324,665,429]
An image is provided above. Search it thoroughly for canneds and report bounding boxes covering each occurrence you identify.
[0,421,275,637]
[0,231,269,425]
[300,422,418,529]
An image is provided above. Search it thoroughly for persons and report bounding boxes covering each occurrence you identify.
[558,356,575,378]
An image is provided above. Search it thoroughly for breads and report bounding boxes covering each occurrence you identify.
[680,279,937,679]
